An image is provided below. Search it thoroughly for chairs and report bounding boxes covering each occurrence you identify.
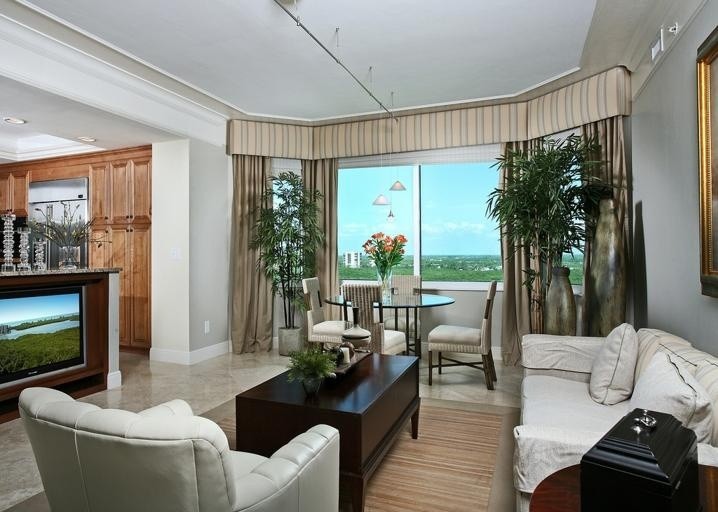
[383,275,422,359]
[428,280,497,390]
[341,280,406,355]
[302,277,347,354]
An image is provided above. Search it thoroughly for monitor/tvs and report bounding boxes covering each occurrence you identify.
[0,283,89,390]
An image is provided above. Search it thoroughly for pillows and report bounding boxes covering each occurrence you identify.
[632,352,715,445]
[589,322,639,406]
[513,328,718,512]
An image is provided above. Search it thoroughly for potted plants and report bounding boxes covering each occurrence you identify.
[287,341,342,392]
[33,200,109,269]
[248,171,326,356]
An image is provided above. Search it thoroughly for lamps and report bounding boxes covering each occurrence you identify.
[372,92,406,222]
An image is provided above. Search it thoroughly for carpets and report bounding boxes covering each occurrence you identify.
[0,397,521,512]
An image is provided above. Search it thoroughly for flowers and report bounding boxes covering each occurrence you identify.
[362,232,408,288]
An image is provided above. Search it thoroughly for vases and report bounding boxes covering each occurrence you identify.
[377,269,392,305]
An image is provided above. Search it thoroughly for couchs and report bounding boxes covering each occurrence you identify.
[18,387,340,512]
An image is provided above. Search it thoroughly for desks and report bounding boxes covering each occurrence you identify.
[324,294,455,356]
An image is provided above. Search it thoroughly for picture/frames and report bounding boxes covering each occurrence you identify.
[696,27,718,298]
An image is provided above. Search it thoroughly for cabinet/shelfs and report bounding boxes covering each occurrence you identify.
[111,227,151,350]
[90,229,108,268]
[91,163,111,225]
[0,171,30,217]
[111,157,152,224]
